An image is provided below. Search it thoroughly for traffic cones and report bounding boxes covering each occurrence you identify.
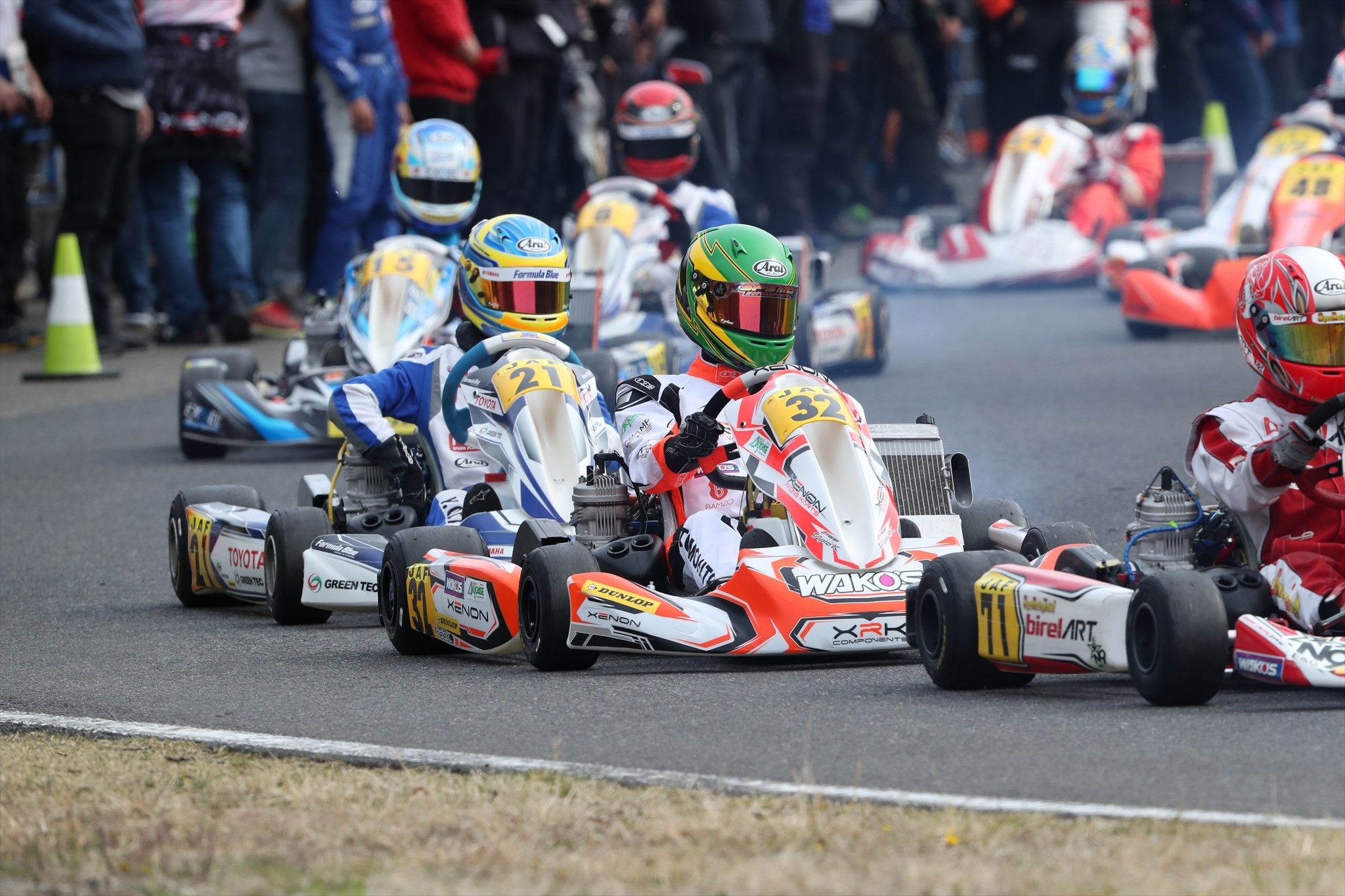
[1201,98,1239,176]
[20,234,119,381]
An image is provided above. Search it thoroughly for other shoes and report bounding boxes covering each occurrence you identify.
[318,340,347,366]
[0,317,47,352]
[116,311,157,348]
[899,517,921,538]
[739,527,779,549]
[459,483,503,521]
[249,295,307,341]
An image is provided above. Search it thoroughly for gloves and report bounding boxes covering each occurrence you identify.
[371,437,428,514]
[675,411,725,461]
[1270,418,1327,473]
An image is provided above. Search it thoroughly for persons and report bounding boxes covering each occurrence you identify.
[1184,245,1345,636]
[327,212,626,528]
[614,223,920,591]
[0,0,1345,365]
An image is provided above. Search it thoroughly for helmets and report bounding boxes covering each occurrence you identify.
[613,79,699,182]
[1233,244,1345,404]
[390,118,483,235]
[1057,33,1141,127]
[458,214,573,338]
[675,223,799,370]
